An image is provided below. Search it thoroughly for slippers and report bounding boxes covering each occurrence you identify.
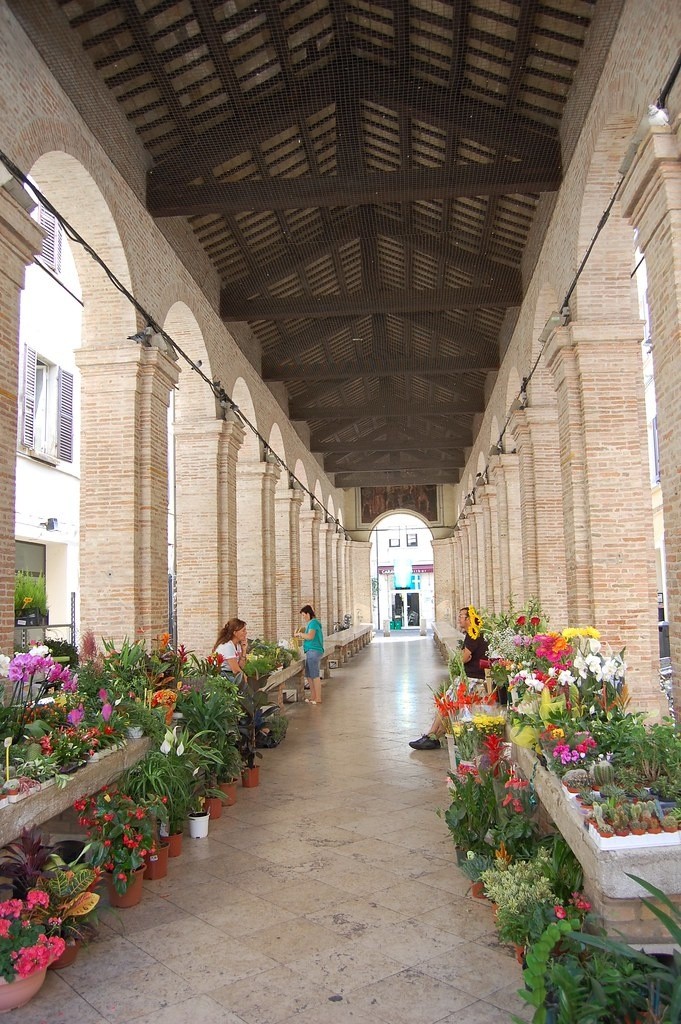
[303,697,323,705]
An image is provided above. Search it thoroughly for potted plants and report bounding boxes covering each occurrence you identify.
[1,634,309,1016]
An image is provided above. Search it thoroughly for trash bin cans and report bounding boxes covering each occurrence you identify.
[389,621,394,630]
[395,621,401,630]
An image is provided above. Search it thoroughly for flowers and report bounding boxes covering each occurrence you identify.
[426,592,681,1024]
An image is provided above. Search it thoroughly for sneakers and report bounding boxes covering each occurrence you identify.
[408,734,441,750]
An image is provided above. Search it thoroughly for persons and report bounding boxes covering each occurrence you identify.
[211,618,275,737]
[294,604,325,705]
[409,607,489,750]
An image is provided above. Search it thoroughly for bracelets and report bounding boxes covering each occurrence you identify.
[240,654,247,661]
